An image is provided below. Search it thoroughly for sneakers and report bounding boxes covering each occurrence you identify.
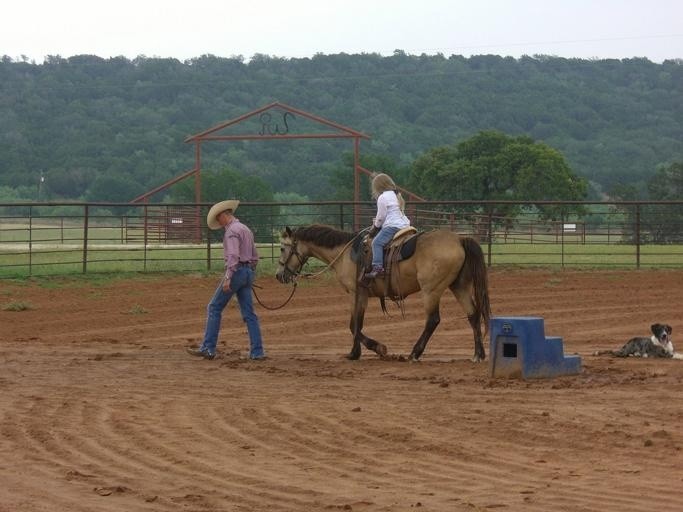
[365,267,384,278]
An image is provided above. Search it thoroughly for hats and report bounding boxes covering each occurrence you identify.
[207,199,240,230]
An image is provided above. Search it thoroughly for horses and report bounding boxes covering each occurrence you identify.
[274,222,493,364]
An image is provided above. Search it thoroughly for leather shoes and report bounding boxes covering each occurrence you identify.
[188,348,214,360]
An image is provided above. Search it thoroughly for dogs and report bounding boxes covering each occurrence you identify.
[592,322,683,360]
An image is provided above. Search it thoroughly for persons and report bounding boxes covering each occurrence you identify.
[186,199,268,361]
[364,171,411,278]
